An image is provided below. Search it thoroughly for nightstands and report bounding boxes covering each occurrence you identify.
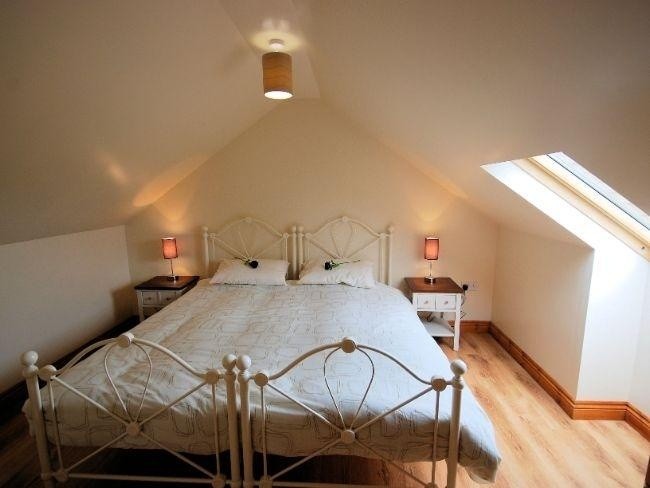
[136,275,198,324]
[406,277,464,350]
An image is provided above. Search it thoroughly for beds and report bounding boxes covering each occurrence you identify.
[21,217,501,487]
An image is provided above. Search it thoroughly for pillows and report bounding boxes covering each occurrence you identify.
[210,248,290,286]
[299,258,377,288]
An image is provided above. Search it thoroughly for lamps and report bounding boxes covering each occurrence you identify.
[424,235,438,282]
[163,238,179,278]
[262,38,292,99]
